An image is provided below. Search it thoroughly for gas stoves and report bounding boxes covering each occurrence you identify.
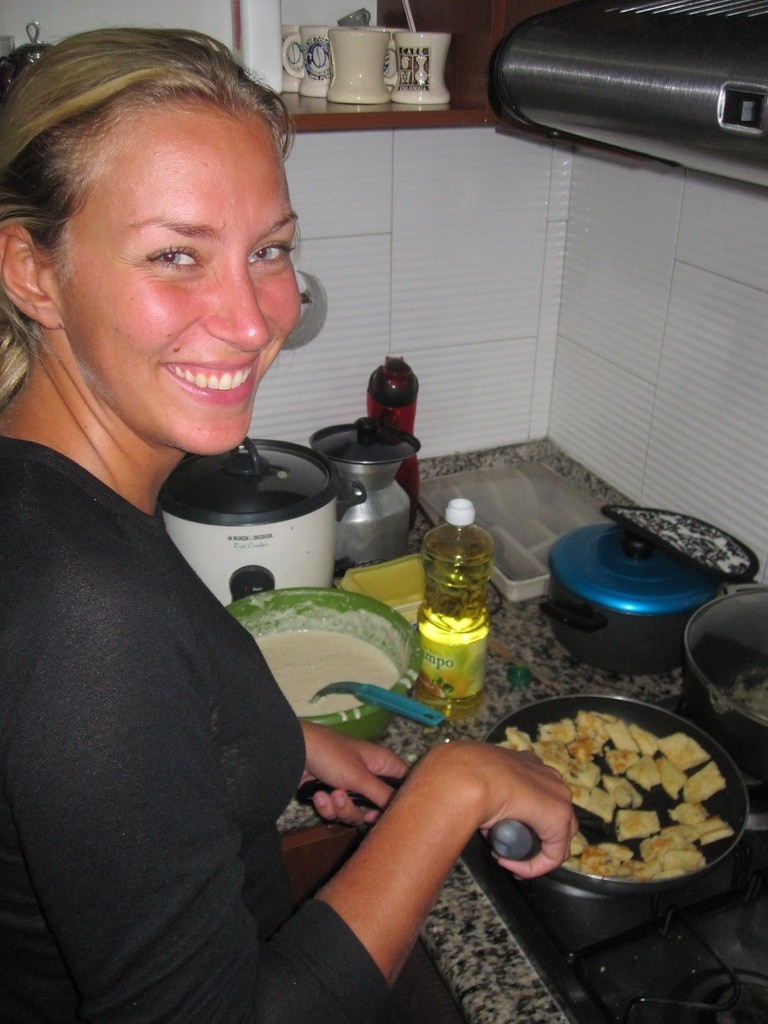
[457,691,768,1024]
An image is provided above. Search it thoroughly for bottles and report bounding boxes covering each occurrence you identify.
[417,499,495,720]
[367,355,420,531]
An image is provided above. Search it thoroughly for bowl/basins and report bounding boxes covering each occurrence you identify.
[336,553,423,626]
[224,587,422,741]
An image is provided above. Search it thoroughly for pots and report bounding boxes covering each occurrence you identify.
[308,417,421,577]
[295,693,749,898]
[158,436,366,611]
[682,583,768,782]
[538,524,724,675]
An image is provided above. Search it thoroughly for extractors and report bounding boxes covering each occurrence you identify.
[486,0,768,188]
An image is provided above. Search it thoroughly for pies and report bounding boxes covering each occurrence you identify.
[493,709,735,881]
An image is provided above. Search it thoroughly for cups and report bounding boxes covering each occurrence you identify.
[283,26,301,93]
[336,8,370,27]
[371,26,408,93]
[390,32,451,105]
[327,29,390,104]
[298,26,330,98]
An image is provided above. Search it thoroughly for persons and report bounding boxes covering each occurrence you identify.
[0,27,578,1024]
